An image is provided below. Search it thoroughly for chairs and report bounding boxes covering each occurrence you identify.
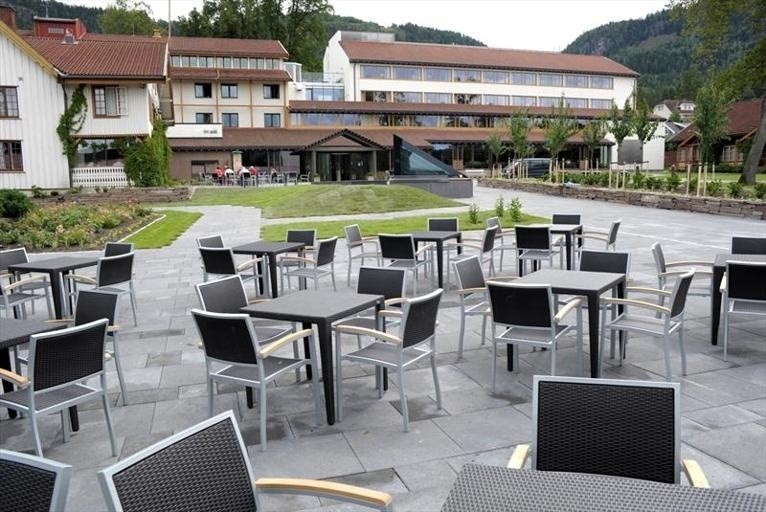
[193,310,323,448]
[100,409,392,512]
[653,242,728,319]
[334,287,442,432]
[199,168,313,187]
[0,451,73,512]
[599,267,696,381]
[191,213,629,391]
[712,237,766,361]
[0,242,137,461]
[507,374,709,492]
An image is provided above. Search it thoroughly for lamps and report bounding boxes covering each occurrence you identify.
[199,168,313,187]
[0,451,73,512]
[507,374,709,492]
[100,409,392,512]
[0,242,137,461]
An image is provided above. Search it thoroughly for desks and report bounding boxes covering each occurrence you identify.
[241,289,388,425]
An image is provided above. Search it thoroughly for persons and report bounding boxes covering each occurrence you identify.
[269,167,277,182]
[215,165,257,184]
